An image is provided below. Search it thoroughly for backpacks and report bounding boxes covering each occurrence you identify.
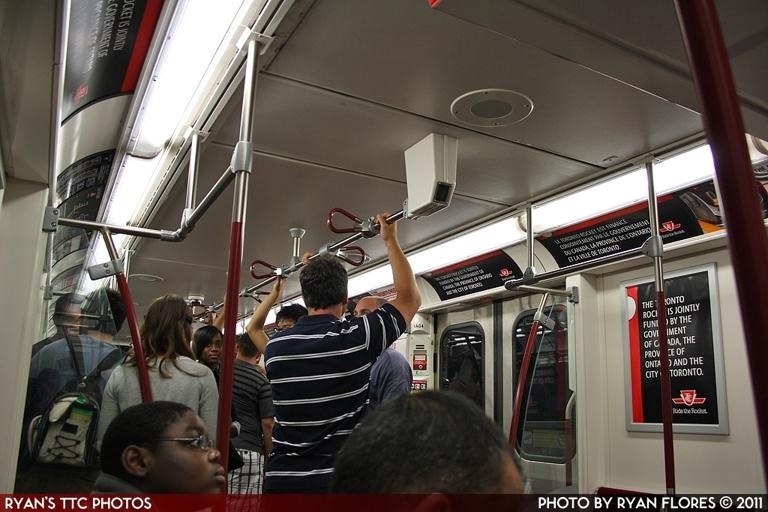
[27,333,126,469]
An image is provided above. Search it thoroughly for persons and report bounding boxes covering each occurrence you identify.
[14,213,527,511]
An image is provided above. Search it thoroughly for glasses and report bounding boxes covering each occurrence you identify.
[146,435,215,452]
